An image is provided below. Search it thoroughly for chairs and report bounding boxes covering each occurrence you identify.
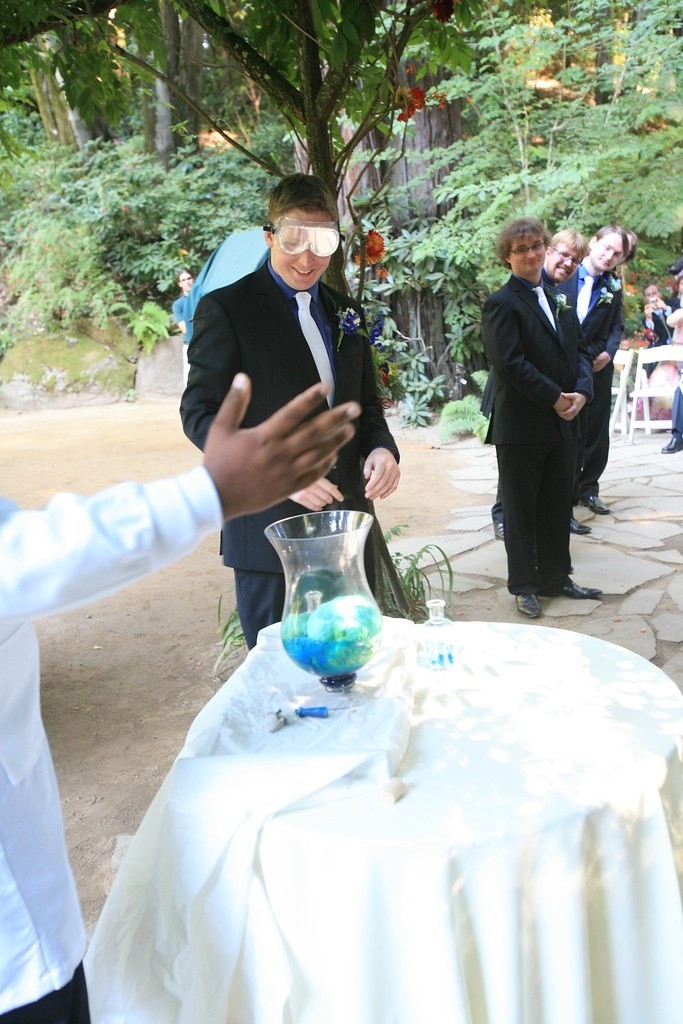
[630,344,683,440]
[609,349,634,437]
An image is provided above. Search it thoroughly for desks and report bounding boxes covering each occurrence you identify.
[85,615,683,1024]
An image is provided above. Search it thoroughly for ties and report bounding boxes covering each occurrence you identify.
[576,276,594,324]
[532,286,556,331]
[295,291,336,410]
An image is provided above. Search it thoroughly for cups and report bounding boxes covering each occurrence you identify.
[263,510,383,711]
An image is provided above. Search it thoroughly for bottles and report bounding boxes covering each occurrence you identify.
[420,598,461,670]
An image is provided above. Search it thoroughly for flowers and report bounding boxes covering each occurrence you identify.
[336,307,370,354]
[596,287,614,307]
[554,293,573,321]
[610,275,622,292]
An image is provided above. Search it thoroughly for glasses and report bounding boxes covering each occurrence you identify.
[553,247,583,269]
[180,276,192,281]
[263,217,346,258]
[508,242,546,257]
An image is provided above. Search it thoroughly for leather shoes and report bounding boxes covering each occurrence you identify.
[493,521,505,541]
[538,576,603,599]
[570,517,592,534]
[661,438,683,454]
[578,495,609,514]
[515,592,541,618]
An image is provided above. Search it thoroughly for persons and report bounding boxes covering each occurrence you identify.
[178,173,400,653]
[0,374,361,1024]
[479,216,637,618]
[171,269,195,389]
[642,255,683,454]
